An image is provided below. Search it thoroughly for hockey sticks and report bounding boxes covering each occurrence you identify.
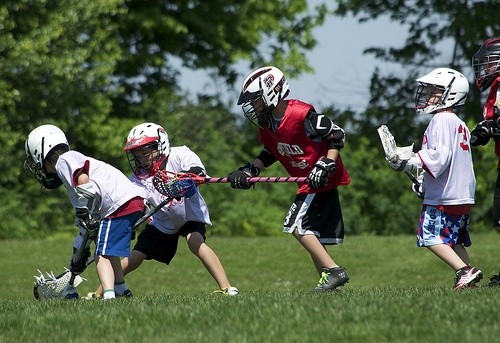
[33,196,174,302]
[376,125,421,188]
[151,170,311,199]
[85,171,206,268]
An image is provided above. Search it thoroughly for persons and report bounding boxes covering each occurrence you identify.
[23,125,146,301]
[227,67,350,293]
[80,122,238,297]
[385,36,500,290]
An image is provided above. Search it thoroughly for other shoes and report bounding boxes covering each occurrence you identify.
[214,287,240,297]
[115,289,133,298]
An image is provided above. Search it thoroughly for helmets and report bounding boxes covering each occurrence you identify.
[414,68,469,116]
[473,38,500,92]
[124,123,170,180]
[23,125,71,170]
[242,66,291,113]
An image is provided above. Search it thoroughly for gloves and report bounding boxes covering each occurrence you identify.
[411,177,426,199]
[470,120,498,147]
[307,156,336,189]
[227,162,261,190]
[75,206,100,232]
[170,166,207,201]
[385,152,412,174]
[69,247,91,275]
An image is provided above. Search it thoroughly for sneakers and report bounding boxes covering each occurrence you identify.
[453,265,483,290]
[314,266,350,291]
